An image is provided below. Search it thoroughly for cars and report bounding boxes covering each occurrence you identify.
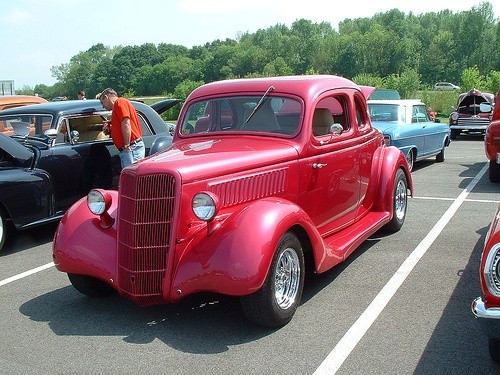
[434,82,460,91]
[450,88,495,142]
[0,99,181,255]
[363,89,401,121]
[53,75,413,327]
[0,95,67,138]
[484,91,500,184]
[366,100,451,172]
[470,202,500,358]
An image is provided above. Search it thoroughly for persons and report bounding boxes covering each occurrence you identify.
[427,107,436,121]
[99,88,145,169]
[57,91,102,143]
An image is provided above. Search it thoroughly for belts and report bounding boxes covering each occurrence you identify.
[119,138,142,153]
